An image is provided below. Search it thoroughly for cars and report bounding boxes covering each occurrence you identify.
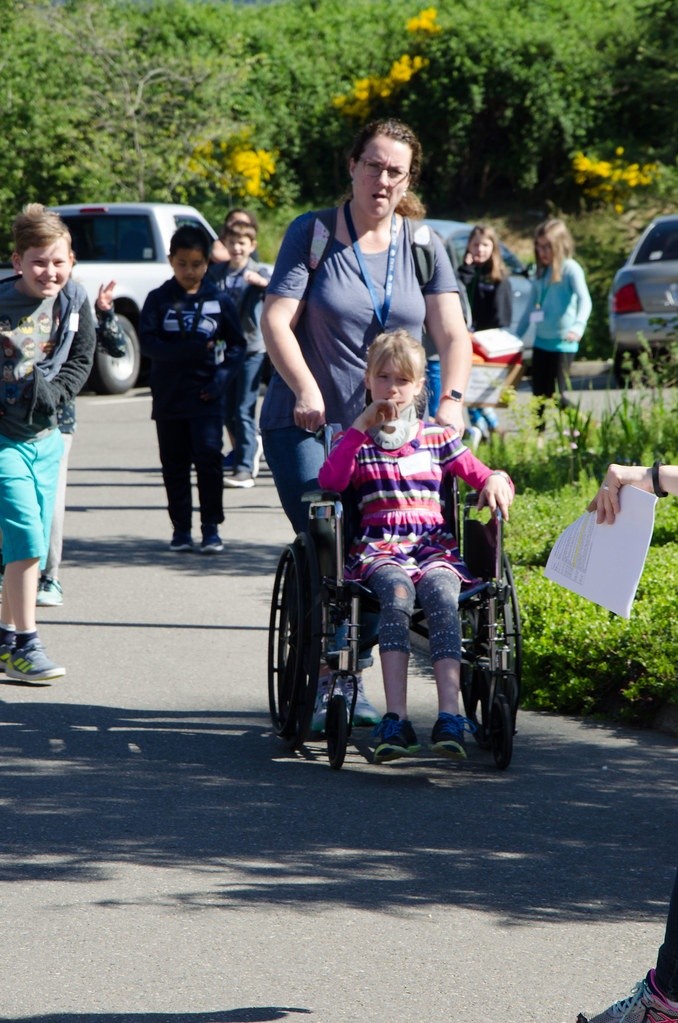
[423,219,538,339]
[605,213,678,391]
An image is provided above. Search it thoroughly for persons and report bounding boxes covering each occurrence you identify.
[207,209,271,488]
[0,214,96,682]
[576,460,678,1023]
[138,225,247,554]
[318,330,515,764]
[0,203,115,608]
[515,219,593,436]
[422,230,472,424]
[457,224,514,332]
[260,122,473,735]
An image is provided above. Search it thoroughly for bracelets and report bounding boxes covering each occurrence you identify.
[652,460,668,497]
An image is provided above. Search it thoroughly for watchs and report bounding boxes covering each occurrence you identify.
[439,389,464,403]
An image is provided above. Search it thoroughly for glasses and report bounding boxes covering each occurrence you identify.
[536,243,551,251]
[359,157,411,180]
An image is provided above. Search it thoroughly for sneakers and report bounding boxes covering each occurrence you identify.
[311,667,351,731]
[6,638,66,681]
[226,470,254,489]
[0,642,15,670]
[36,575,63,607]
[338,676,380,724]
[168,530,194,551]
[428,712,476,759]
[248,432,264,478]
[591,972,678,1023]
[370,712,421,761]
[200,534,224,553]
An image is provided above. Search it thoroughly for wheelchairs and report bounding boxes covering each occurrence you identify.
[268,418,525,770]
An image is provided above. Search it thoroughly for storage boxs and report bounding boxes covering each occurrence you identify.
[463,327,522,410]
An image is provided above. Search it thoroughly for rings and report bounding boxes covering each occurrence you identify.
[602,485,609,491]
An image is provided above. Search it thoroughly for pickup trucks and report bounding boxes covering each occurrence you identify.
[1,201,276,395]
